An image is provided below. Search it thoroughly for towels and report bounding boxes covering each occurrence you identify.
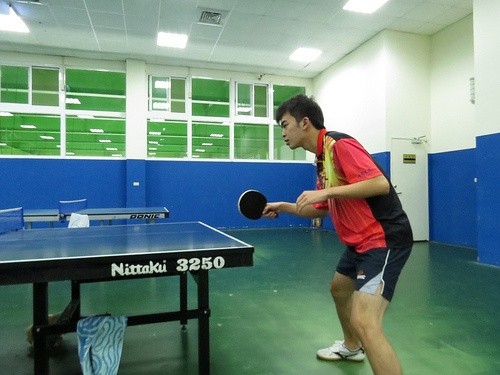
[76,314,128,375]
[69,212,90,228]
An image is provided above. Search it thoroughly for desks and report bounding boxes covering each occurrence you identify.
[0,207,256,375]
[0,198,170,231]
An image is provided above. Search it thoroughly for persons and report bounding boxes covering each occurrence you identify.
[261,94,414,375]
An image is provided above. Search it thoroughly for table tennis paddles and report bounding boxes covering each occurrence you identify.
[238,189,278,220]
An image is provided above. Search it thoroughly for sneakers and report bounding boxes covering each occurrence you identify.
[316,340,366,362]
